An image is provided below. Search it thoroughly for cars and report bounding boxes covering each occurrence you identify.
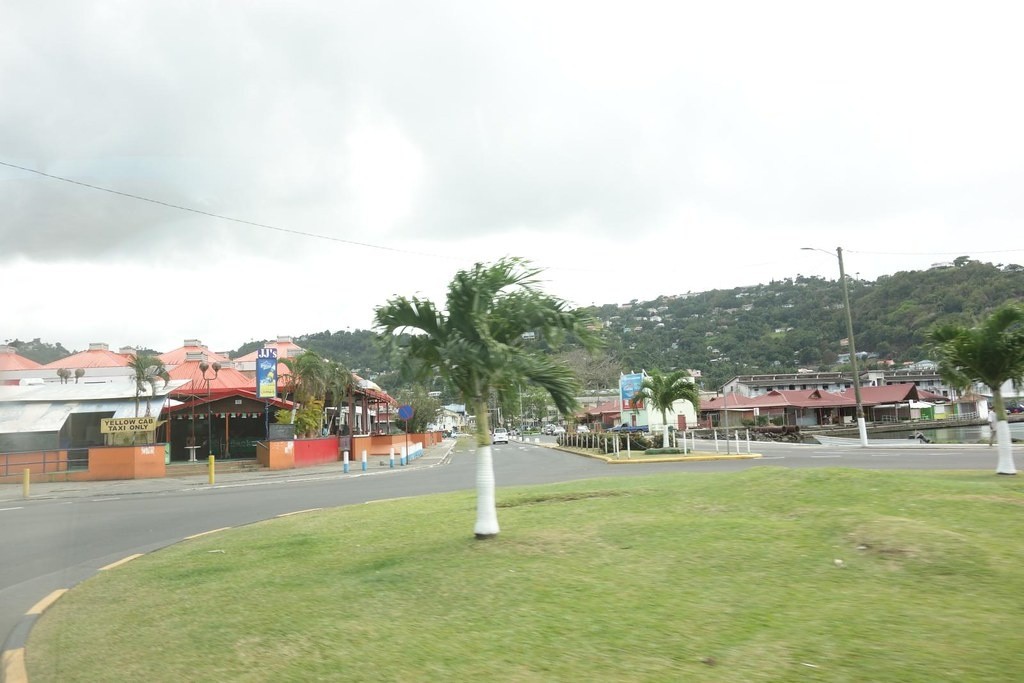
[542,424,567,436]
[491,427,509,444]
[835,358,852,365]
[605,426,614,434]
[574,425,590,434]
[450,432,469,439]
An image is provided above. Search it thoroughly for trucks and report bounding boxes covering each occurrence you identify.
[613,421,649,435]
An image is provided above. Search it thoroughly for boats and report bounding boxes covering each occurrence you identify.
[908,428,934,444]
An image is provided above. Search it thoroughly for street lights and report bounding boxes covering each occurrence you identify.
[199,362,222,454]
[800,246,868,447]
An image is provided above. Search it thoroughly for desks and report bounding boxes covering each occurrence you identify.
[184,445,202,462]
